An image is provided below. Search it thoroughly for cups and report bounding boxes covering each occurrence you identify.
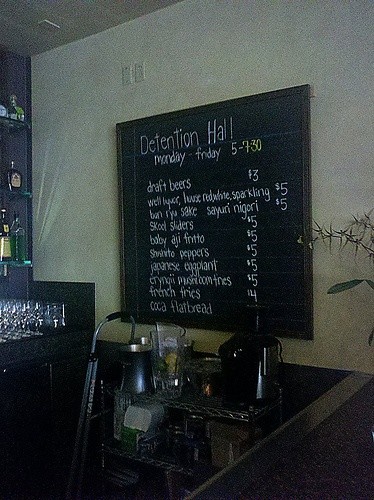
[42,302,66,329]
[148,322,187,399]
[121,344,155,395]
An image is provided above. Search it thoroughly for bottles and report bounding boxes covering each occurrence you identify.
[0,208,25,262]
[113,391,132,440]
[0,93,18,120]
[6,160,22,192]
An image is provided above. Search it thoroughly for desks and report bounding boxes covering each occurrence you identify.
[185,374,374,500]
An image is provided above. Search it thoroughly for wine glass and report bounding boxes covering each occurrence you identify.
[0,298,44,344]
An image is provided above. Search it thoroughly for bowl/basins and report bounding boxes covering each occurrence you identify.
[188,359,223,397]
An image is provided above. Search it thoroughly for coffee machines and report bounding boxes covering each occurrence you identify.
[217,304,281,409]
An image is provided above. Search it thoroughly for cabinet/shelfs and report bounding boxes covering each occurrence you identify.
[0,45,33,281]
[0,280,283,500]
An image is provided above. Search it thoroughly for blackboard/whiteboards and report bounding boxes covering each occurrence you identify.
[113,81,316,343]
